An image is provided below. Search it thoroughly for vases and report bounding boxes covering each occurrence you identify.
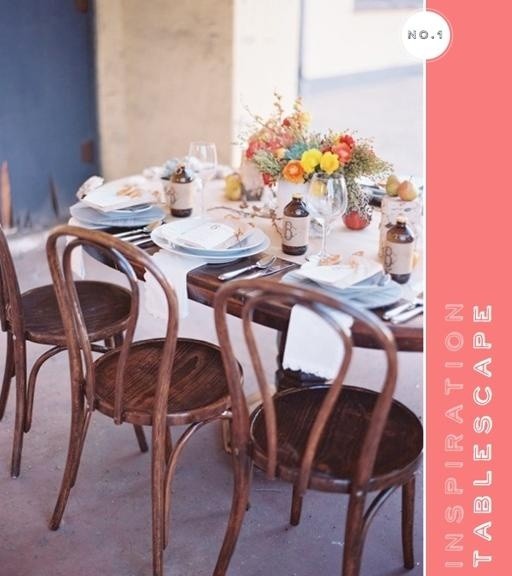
[275,176,308,214]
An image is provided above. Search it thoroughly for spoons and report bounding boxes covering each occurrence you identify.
[381,291,423,321]
[111,219,162,238]
[217,254,277,280]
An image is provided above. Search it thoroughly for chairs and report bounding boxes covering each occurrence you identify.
[1,226,423,576]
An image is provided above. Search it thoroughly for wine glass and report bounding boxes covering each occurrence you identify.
[305,172,348,264]
[187,140,218,220]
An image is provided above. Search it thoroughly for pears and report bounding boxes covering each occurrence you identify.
[398,180,418,201]
[386,174,400,195]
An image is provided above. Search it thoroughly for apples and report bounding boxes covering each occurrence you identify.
[342,210,369,230]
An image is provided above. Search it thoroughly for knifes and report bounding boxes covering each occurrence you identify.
[232,264,296,281]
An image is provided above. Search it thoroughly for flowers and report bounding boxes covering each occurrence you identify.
[242,90,391,186]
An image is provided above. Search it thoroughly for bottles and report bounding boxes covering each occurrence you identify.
[169,163,196,218]
[281,192,310,256]
[384,217,415,286]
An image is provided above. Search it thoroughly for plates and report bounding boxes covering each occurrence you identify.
[359,181,424,202]
[70,199,167,228]
[151,219,271,265]
[281,268,404,310]
